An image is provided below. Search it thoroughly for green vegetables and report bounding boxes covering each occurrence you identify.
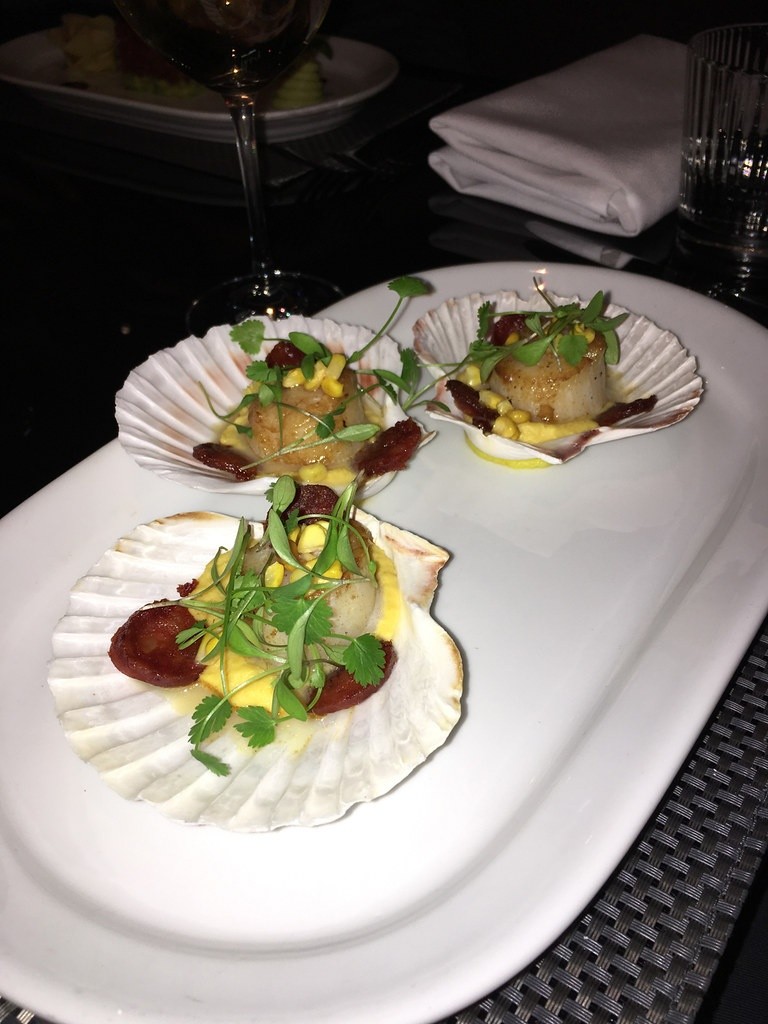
[201,276,630,473]
[138,466,386,777]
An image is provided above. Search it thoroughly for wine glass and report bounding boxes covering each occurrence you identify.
[116,0,348,333]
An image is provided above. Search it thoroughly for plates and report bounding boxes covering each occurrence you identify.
[0,260,768,1024]
[1,22,399,143]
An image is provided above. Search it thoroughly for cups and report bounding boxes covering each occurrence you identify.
[678,21,768,281]
